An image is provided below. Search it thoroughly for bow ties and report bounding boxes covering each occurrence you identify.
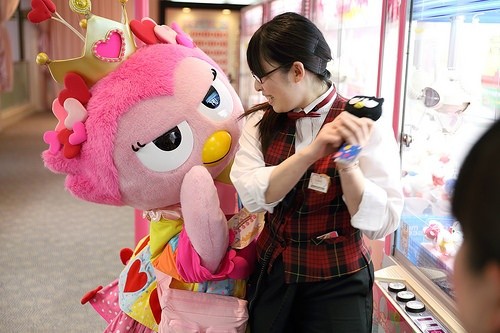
[287,83,336,120]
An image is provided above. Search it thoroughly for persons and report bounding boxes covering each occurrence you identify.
[229,11,401,333]
[449,117,500,333]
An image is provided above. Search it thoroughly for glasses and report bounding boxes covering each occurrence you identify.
[250,62,286,84]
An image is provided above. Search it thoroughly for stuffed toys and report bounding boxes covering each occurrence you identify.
[26,0,255,333]
